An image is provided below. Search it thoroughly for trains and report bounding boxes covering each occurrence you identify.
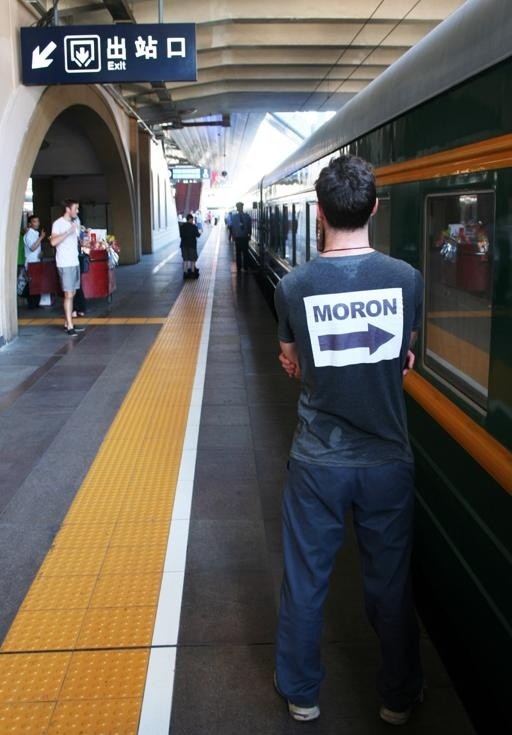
[237,3,511,504]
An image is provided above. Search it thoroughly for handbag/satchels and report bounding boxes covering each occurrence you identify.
[78,253,89,273]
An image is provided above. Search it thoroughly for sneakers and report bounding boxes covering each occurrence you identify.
[63,325,85,337]
[272,668,322,722]
[378,686,425,726]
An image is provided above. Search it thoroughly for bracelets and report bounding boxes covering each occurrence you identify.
[67,228,74,235]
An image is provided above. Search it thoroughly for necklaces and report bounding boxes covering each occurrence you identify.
[319,245,373,255]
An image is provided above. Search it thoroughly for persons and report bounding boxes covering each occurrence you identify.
[177,208,237,243]
[427,222,491,262]
[231,202,253,281]
[21,214,47,310]
[179,213,201,280]
[50,196,87,337]
[270,152,425,726]
